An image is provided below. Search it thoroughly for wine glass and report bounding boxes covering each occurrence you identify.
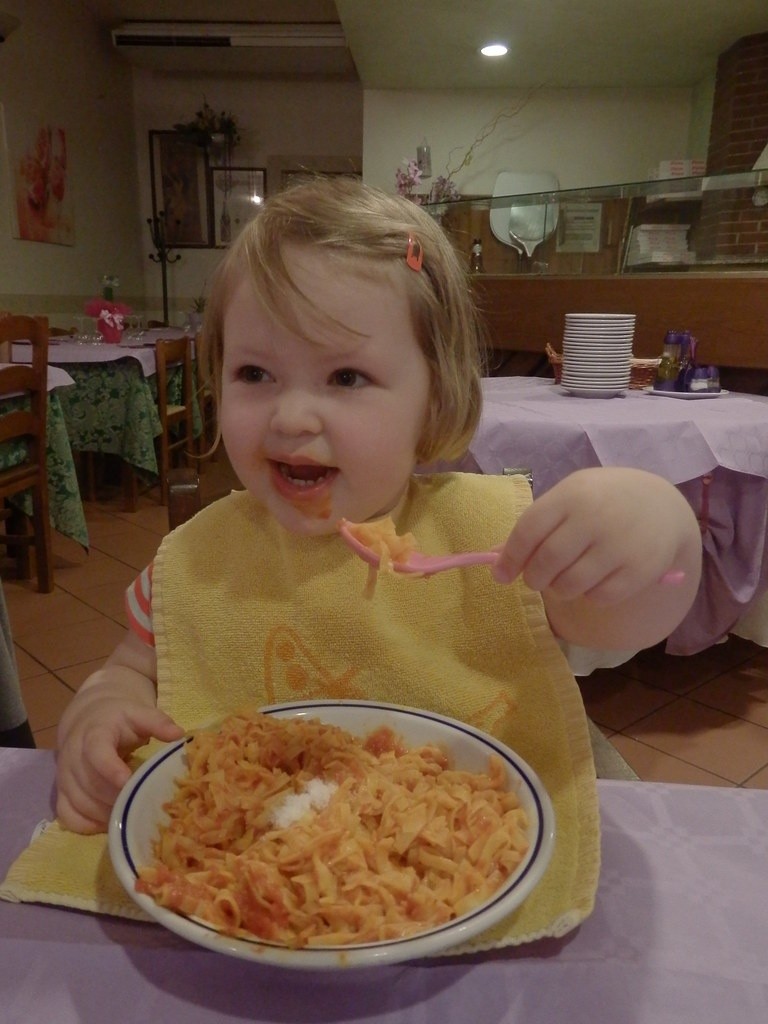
[72,314,105,347]
[122,313,148,347]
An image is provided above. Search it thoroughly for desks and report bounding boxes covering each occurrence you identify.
[11,345,180,506]
[0,747,768,1024]
[57,327,210,457]
[415,377,768,676]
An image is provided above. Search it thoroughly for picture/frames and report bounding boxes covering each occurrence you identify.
[211,167,267,249]
[148,129,212,248]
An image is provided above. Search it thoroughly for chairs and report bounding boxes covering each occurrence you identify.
[193,335,215,449]
[0,314,54,593]
[84,337,194,508]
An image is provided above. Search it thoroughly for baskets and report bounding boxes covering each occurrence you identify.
[545,342,662,388]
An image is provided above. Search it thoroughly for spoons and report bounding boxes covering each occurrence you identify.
[338,517,686,584]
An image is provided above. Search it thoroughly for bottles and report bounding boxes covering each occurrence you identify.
[466,238,486,274]
[657,328,721,392]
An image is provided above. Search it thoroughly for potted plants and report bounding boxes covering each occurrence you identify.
[188,288,205,326]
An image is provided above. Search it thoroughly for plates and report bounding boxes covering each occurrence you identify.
[108,699,556,969]
[642,386,730,400]
[561,313,636,396]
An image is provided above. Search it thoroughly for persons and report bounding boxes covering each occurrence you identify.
[56,175,704,837]
